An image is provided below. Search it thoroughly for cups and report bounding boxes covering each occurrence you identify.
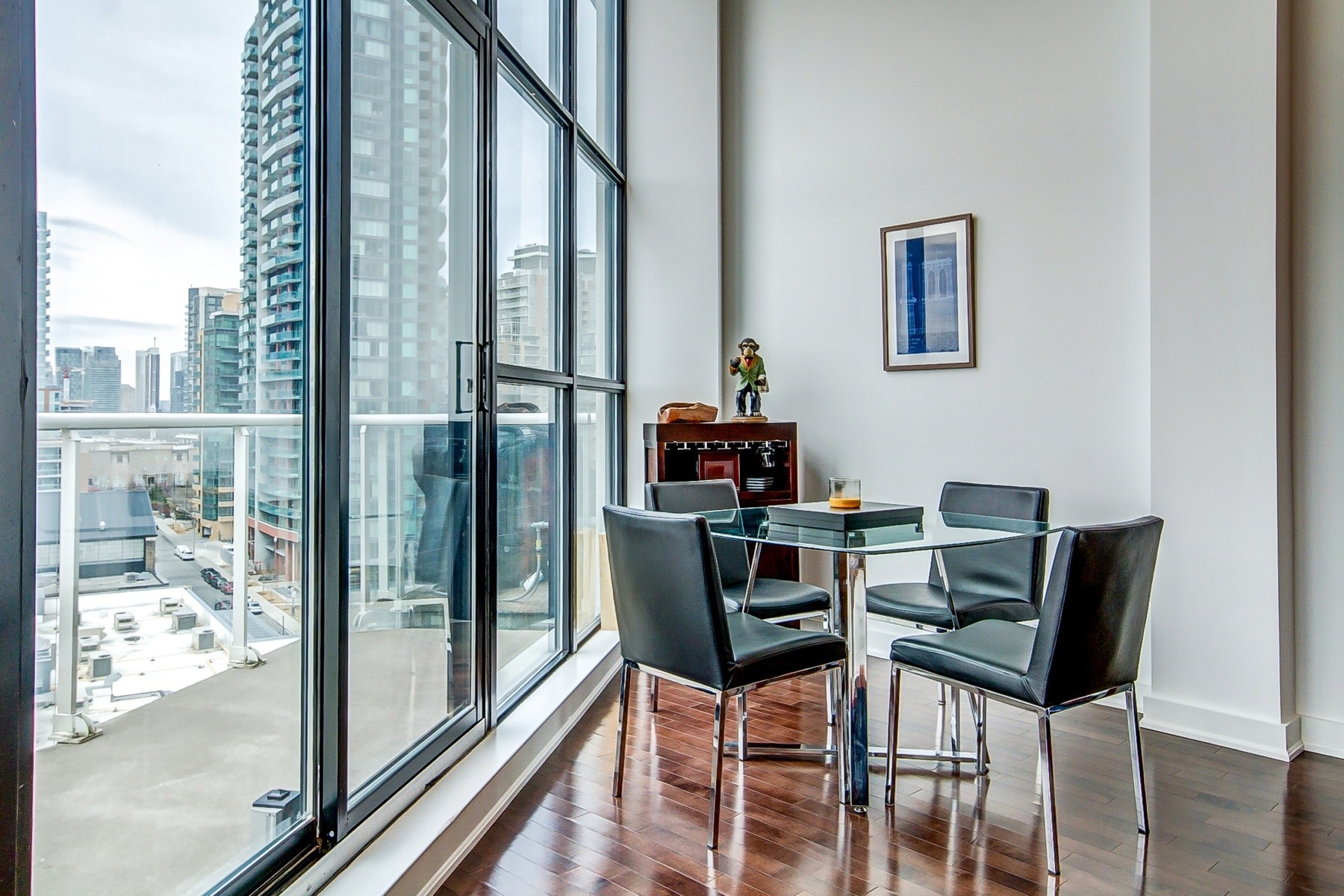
[828,477,861,508]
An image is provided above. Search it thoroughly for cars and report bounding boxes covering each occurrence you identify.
[215,600,233,611]
[201,568,234,595]
[247,601,263,615]
[174,545,195,561]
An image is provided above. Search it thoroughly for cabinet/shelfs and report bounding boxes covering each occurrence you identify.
[639,418,804,633]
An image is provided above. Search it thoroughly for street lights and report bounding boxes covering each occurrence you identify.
[175,507,196,554]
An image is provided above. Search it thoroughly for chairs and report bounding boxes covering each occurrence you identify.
[877,510,1168,876]
[865,478,1054,766]
[589,493,862,850]
[644,475,837,729]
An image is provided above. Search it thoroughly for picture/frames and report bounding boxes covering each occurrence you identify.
[878,211,977,372]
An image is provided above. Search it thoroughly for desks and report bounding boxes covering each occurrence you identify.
[674,502,1070,811]
